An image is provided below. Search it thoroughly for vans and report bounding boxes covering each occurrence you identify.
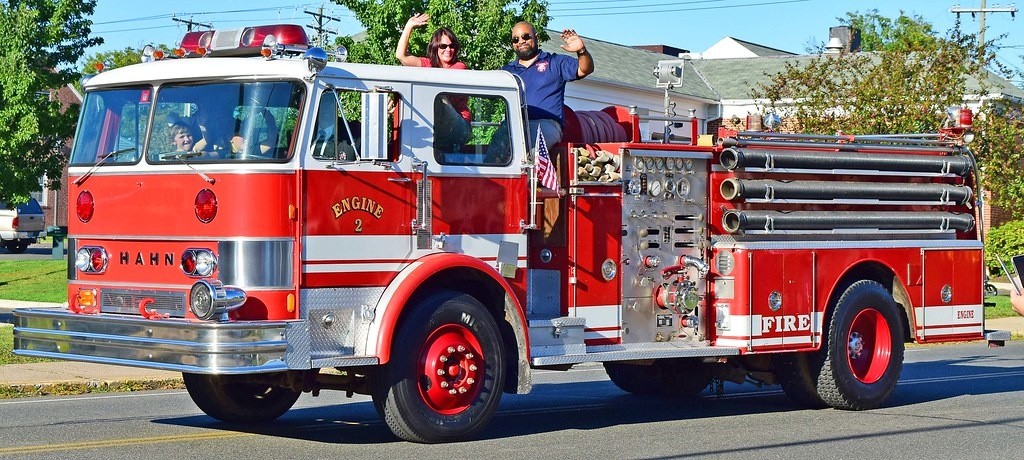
[0,198,47,253]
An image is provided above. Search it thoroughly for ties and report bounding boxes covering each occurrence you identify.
[313,130,326,156]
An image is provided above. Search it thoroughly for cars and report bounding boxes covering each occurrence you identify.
[11,24,1011,444]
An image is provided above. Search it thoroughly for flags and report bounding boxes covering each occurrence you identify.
[536,131,562,198]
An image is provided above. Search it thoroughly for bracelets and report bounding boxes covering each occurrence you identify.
[577,48,586,55]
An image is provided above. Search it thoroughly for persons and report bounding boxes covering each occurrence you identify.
[171,104,278,158]
[484,21,595,163]
[396,13,472,143]
[312,97,395,158]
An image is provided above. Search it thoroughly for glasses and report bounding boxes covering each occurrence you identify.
[439,44,454,49]
[511,33,534,43]
